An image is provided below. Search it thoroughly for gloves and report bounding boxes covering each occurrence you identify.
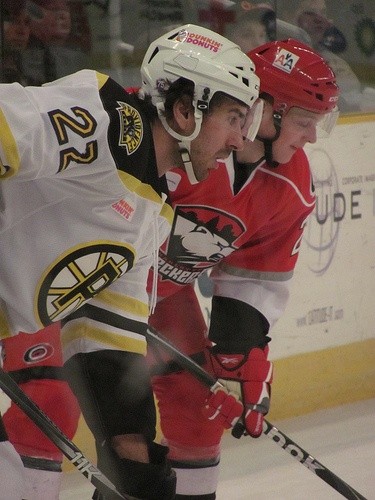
[92,437,177,500]
[204,332,273,438]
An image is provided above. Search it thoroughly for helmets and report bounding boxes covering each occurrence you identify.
[246,38,340,114]
[139,24,260,108]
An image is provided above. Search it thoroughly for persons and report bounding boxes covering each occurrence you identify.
[213,0,375,116]
[0,38,340,500]
[0,0,74,87]
[0,23,260,500]
